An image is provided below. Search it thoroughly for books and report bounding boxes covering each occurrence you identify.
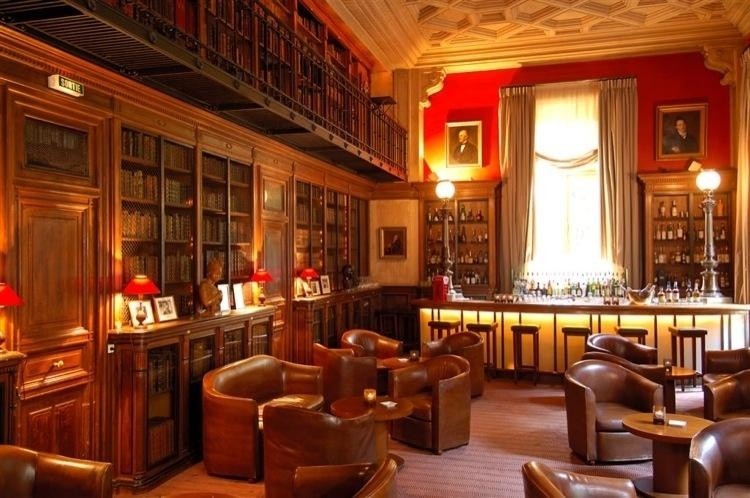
[297,183,358,272]
[149,325,268,396]
[123,132,250,283]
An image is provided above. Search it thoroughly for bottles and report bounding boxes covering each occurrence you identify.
[423,202,489,285]
[512,267,626,298]
[640,283,655,292]
[653,197,731,304]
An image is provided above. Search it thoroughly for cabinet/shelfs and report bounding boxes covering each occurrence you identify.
[308,288,381,348]
[220,308,273,366]
[294,174,369,298]
[636,168,737,298]
[113,118,256,315]
[410,180,503,288]
[108,324,224,495]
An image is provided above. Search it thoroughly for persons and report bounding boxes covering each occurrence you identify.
[384,234,400,254]
[667,118,698,153]
[452,128,478,164]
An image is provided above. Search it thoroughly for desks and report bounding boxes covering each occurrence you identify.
[0,351,28,445]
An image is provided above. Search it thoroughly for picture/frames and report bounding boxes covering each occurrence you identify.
[217,282,245,313]
[445,120,483,167]
[128,295,178,326]
[380,226,407,259]
[655,102,708,161]
[311,275,331,295]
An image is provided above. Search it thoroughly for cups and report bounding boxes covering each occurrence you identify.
[493,294,517,303]
[603,296,620,305]
[650,404,668,425]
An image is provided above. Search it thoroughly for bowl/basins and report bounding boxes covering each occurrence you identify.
[628,290,654,304]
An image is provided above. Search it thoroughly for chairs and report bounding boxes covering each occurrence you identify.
[0,443,113,498]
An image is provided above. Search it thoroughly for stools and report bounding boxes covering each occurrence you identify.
[467,322,498,382]
[510,324,541,386]
[428,321,461,341]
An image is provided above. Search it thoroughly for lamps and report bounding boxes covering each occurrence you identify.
[251,268,273,307]
[695,169,725,299]
[300,268,319,298]
[435,181,466,298]
[123,275,160,329]
[0,283,25,355]
